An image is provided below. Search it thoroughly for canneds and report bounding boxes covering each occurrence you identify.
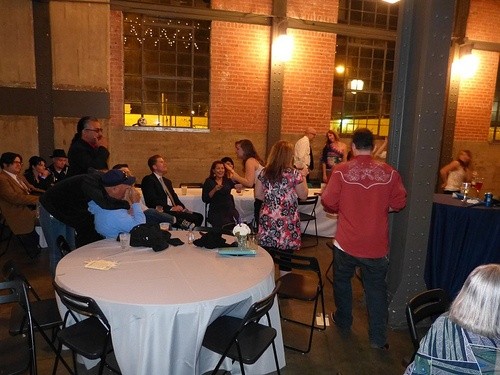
[484,192,493,207]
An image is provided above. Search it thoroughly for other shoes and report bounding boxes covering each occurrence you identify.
[331,312,336,323]
[372,343,390,352]
[172,217,196,231]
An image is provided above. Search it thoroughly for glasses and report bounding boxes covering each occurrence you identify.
[85,128,103,133]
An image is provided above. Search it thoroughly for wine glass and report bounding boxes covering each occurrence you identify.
[460,182,472,205]
[474,178,484,199]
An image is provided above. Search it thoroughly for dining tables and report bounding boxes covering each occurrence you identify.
[53,230,286,375]
[425,193,500,300]
[131,186,256,232]
[295,188,341,237]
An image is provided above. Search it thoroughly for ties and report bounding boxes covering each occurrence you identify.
[160,177,176,206]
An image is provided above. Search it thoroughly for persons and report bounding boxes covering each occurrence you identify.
[228,140,266,233]
[140,155,204,231]
[55,116,137,247]
[202,161,240,230]
[255,141,308,278]
[292,127,316,183]
[440,150,476,195]
[112,163,195,231]
[321,130,347,184]
[221,157,234,169]
[0,152,45,235]
[404,264,500,375]
[321,129,407,352]
[45,149,69,184]
[23,156,50,196]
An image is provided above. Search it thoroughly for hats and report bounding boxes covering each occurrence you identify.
[49,149,68,159]
[102,169,136,186]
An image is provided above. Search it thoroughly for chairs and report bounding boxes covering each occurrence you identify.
[404,288,449,367]
[297,196,318,249]
[266,248,326,352]
[200,281,283,375]
[52,281,123,375]
[0,281,37,375]
[204,201,212,227]
[5,262,77,375]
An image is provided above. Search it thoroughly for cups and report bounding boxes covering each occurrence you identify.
[188,232,195,244]
[160,222,170,232]
[247,232,259,249]
[119,233,130,249]
[234,231,247,249]
[181,186,187,195]
[321,183,326,192]
[235,184,242,193]
